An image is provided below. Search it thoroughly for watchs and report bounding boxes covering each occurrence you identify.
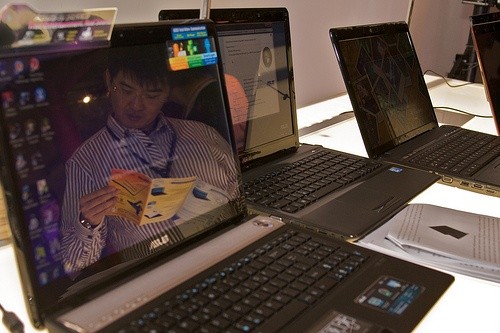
[80,212,101,230]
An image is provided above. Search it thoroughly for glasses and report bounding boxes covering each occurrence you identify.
[111,80,168,101]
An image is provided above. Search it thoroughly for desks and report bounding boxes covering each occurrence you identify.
[0,74,500,333]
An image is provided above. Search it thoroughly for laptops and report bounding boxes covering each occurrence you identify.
[1,19,454,333]
[157,8,440,242]
[329,21,500,200]
[468,11,500,131]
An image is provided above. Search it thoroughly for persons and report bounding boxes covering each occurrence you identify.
[60,47,243,274]
